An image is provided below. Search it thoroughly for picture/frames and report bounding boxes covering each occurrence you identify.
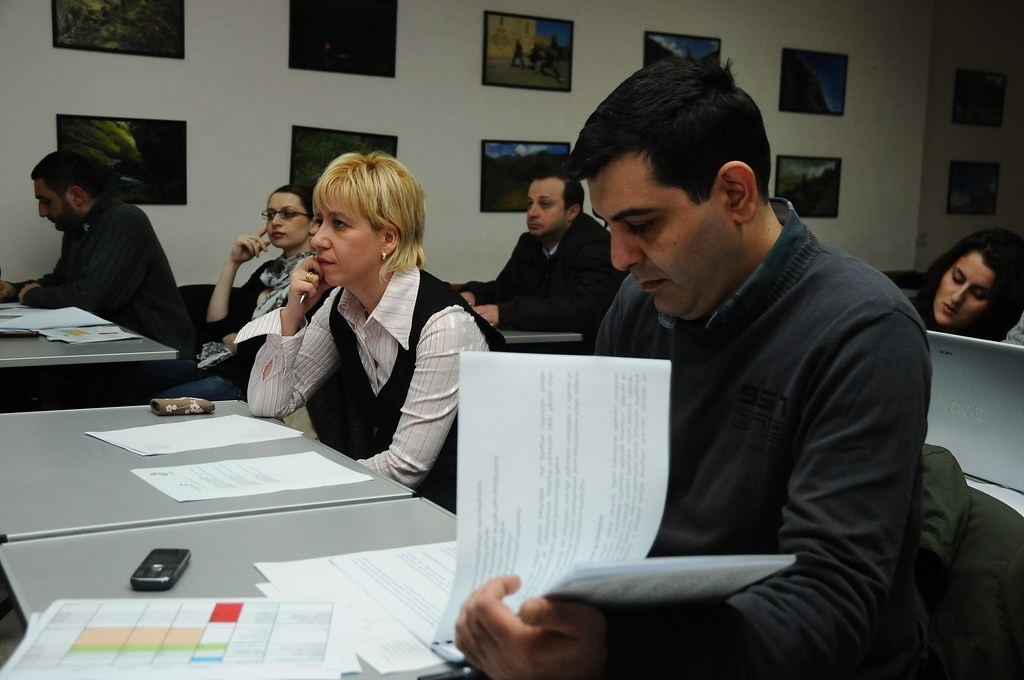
[947,161,999,215]
[775,155,841,218]
[51,0,185,58]
[289,124,397,194]
[952,69,1007,127]
[479,139,571,212]
[481,10,574,92]
[643,31,721,69]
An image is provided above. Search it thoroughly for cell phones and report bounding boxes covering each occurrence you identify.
[130,548,191,591]
[0,330,39,337]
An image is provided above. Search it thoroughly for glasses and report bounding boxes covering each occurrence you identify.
[260,208,312,221]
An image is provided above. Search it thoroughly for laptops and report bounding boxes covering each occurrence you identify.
[924,330,1024,494]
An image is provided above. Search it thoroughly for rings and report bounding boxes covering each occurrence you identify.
[305,272,314,281]
[248,237,252,242]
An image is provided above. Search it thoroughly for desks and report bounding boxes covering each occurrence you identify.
[0,296,582,680]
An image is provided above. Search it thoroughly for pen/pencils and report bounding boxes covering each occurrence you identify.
[298,292,309,305]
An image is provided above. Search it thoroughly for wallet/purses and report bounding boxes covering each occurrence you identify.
[150,397,215,416]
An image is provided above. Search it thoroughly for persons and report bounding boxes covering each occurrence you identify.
[0,151,197,409]
[909,228,1024,346]
[142,185,317,401]
[457,163,630,332]
[455,57,933,680]
[247,150,507,489]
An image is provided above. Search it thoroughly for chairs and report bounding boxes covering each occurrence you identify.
[923,482,1024,680]
[181,283,241,354]
